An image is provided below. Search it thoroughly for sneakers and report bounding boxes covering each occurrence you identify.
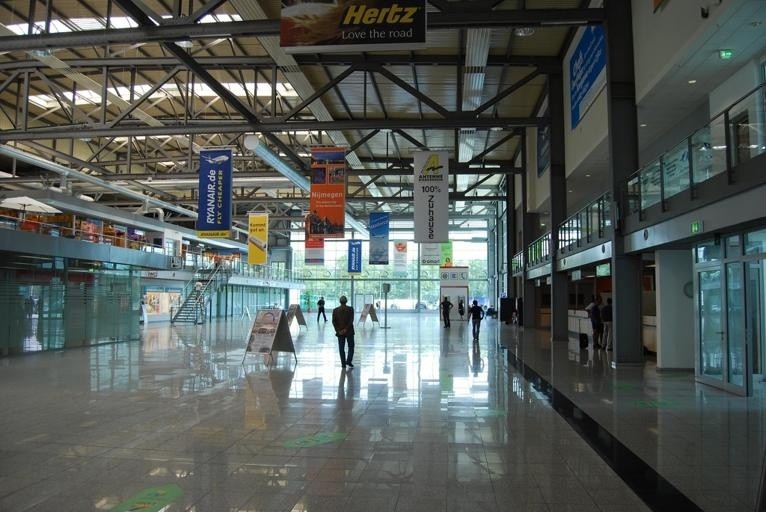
[342,362,353,367]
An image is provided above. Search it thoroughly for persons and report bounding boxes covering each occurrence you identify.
[317,297,328,322]
[467,339,484,377]
[441,328,453,357]
[470,300,484,340]
[335,367,355,432]
[332,296,355,367]
[440,297,453,328]
[317,322,327,344]
[458,300,464,320]
[585,296,613,351]
[195,280,202,297]
